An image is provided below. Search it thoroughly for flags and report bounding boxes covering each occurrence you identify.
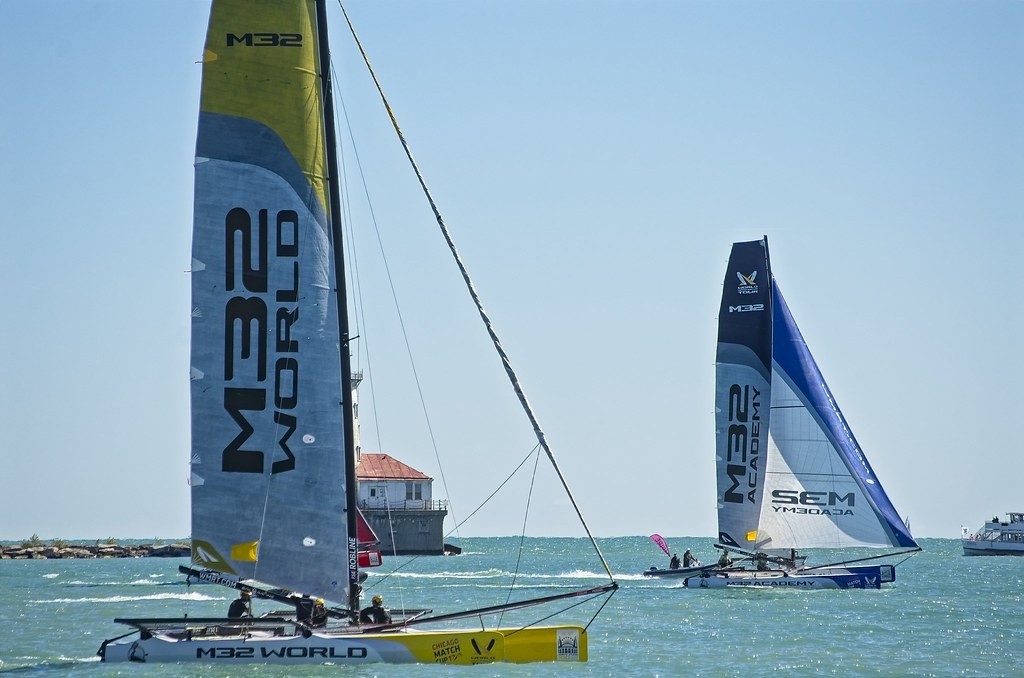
[961,527,970,536]
[747,531,757,541]
[231,540,260,563]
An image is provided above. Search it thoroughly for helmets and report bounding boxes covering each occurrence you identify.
[372,595,382,604]
[241,590,252,597]
[316,598,324,604]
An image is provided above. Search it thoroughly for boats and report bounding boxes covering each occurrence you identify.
[961,512,1024,556]
[643,534,718,580]
[355,454,449,556]
[444,544,461,554]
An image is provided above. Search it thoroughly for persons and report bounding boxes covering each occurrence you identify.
[349,595,392,625]
[969,534,981,540]
[312,599,328,627]
[690,558,702,568]
[684,548,695,567]
[992,516,999,523]
[757,561,771,571]
[228,589,254,626]
[670,553,680,569]
[718,550,731,564]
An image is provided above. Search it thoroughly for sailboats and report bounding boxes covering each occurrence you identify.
[97,0,620,666]
[683,235,922,590]
[356,507,383,567]
